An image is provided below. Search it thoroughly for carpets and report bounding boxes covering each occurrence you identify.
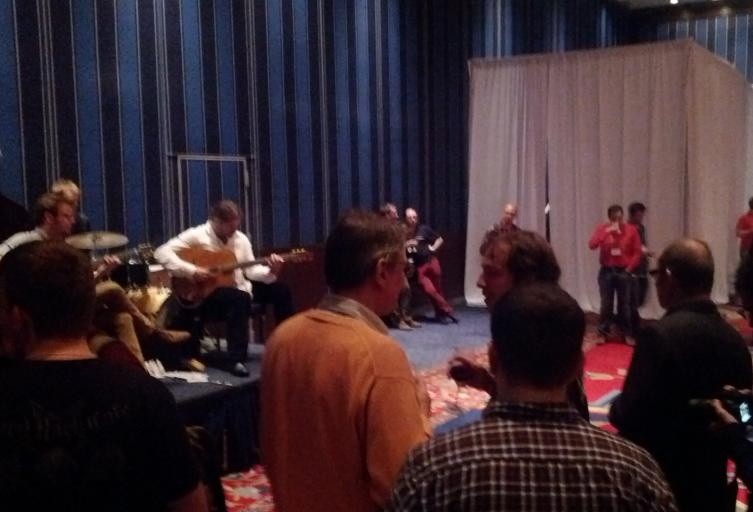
[220,322,753,512]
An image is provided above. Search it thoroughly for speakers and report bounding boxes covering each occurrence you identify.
[108,262,148,286]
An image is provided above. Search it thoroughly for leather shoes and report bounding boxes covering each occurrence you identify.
[451,314,461,324]
[224,358,249,377]
[624,337,635,346]
[397,321,411,330]
[596,335,606,346]
[437,315,450,325]
[407,319,421,328]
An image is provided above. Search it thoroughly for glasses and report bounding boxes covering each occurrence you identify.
[649,267,671,279]
[388,260,417,281]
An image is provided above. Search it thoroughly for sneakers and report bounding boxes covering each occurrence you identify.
[157,328,191,347]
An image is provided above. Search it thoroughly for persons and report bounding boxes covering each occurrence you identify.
[388,281,679,512]
[0,178,192,376]
[735,196,753,315]
[0,241,210,512]
[588,204,641,346]
[615,202,657,327]
[378,200,423,332]
[607,238,753,511]
[258,210,429,512]
[153,198,286,378]
[448,225,590,422]
[403,207,460,326]
[476,203,520,288]
[708,385,753,512]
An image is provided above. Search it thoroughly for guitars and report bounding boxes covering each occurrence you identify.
[169,246,313,310]
[94,243,154,279]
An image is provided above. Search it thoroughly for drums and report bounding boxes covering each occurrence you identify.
[126,288,170,329]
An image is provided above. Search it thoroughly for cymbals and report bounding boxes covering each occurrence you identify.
[65,233,128,248]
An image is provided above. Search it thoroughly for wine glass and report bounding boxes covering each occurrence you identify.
[446,344,469,415]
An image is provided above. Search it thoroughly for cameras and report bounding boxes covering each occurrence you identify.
[405,245,418,265]
[686,389,751,429]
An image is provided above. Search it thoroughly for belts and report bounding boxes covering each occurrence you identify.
[600,264,630,275]
